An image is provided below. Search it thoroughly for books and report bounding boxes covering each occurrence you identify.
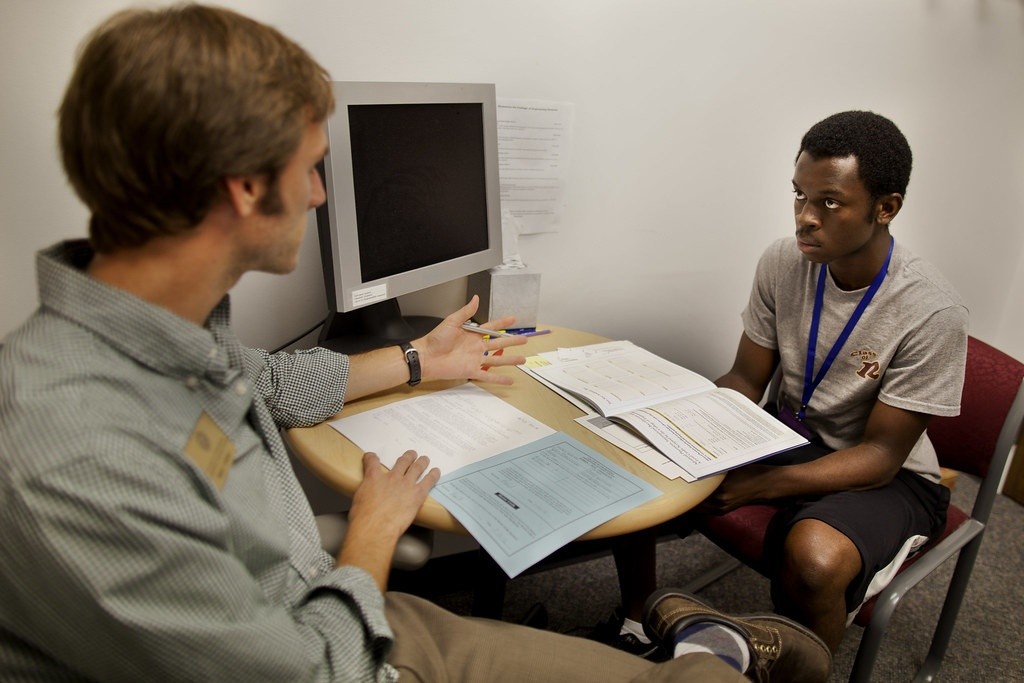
[531,346,811,478]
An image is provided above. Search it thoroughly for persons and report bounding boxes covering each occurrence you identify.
[713,111,969,659]
[0,3,834,683]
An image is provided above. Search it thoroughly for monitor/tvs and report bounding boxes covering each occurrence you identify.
[314,81,504,313]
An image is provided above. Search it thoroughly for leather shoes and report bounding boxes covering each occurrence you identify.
[643,588,833,683]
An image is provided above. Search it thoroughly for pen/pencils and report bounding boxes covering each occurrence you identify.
[516,329,551,338]
[460,321,513,339]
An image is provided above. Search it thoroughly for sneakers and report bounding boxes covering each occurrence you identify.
[561,608,673,664]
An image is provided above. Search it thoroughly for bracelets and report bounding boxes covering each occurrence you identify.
[399,343,422,387]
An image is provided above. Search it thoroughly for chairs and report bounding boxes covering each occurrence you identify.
[702,333,1024,683]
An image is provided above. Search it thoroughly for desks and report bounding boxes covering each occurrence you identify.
[277,323,729,614]
